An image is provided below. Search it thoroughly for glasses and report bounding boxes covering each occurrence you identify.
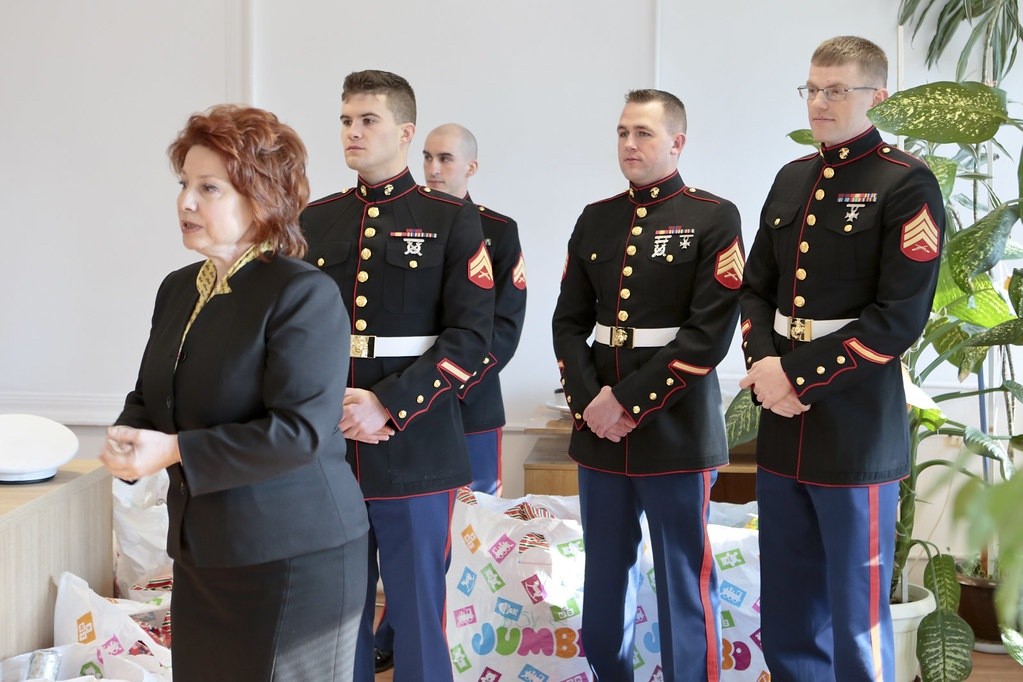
[797,84,878,102]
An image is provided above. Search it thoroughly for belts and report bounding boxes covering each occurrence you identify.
[773,308,860,342]
[594,322,680,349]
[349,335,440,358]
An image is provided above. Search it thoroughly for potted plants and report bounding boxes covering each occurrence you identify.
[728,81,1023,682]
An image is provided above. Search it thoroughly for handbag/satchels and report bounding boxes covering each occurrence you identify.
[1,466,173,681]
[443,489,774,681]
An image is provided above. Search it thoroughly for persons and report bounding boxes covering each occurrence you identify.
[301,71,495,682]
[551,89,744,682]
[370,121,528,673]
[740,37,946,682]
[99,104,370,682]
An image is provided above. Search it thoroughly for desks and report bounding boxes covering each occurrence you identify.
[523,438,757,499]
[0,459,113,661]
